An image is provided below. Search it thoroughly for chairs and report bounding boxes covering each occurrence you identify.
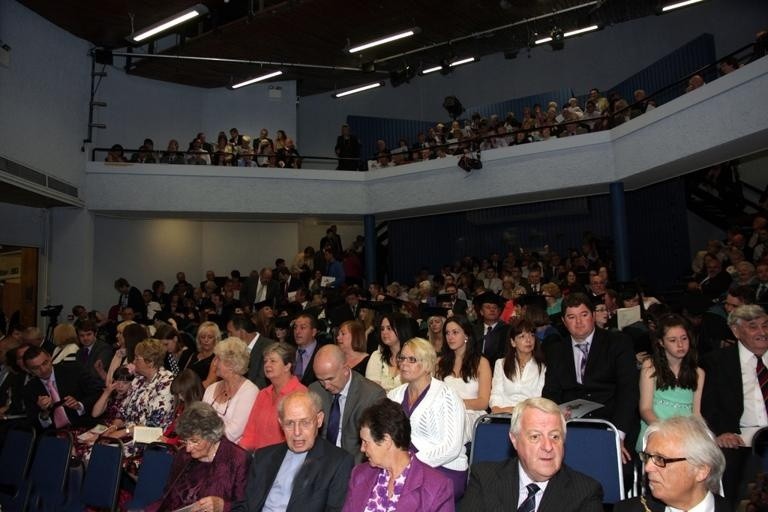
[0,376,627,511]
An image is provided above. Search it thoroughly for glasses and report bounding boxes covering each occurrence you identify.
[638,450,687,469]
[178,436,206,449]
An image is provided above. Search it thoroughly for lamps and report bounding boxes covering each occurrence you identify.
[121,1,607,99]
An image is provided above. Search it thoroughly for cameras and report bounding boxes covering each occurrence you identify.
[47,399,65,410]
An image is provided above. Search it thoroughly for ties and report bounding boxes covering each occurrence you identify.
[754,354,768,418]
[574,342,590,382]
[758,285,767,299]
[293,348,307,377]
[516,483,540,512]
[43,380,70,429]
[325,392,344,446]
[81,348,90,368]
[483,326,493,353]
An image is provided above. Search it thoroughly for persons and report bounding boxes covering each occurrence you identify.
[687,75,705,92]
[372,88,656,171]
[752,30,768,61]
[719,56,745,77]
[1,224,768,510]
[105,128,303,169]
[335,123,358,170]
